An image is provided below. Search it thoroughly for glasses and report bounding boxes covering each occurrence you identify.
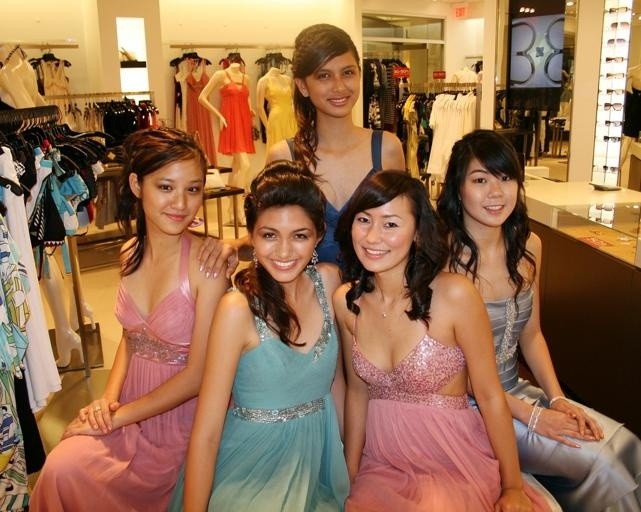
[596,204,614,211]
[611,22,630,30]
[606,72,626,80]
[603,166,620,173]
[596,218,614,225]
[606,57,625,65]
[604,102,624,111]
[610,6,629,15]
[603,135,621,143]
[608,39,627,46]
[606,88,625,96]
[605,120,624,128]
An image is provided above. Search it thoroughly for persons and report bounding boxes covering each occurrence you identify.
[164,160,350,511]
[258,67,299,160]
[332,169,554,512]
[199,63,258,226]
[620,64,641,168]
[25,124,237,512]
[194,23,406,281]
[36,246,98,367]
[436,127,641,512]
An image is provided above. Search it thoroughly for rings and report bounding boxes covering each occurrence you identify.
[93,406,101,411]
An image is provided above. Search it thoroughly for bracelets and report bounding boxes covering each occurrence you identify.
[528,404,544,432]
[550,396,568,407]
[504,478,526,490]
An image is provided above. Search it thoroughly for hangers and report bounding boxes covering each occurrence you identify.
[170,44,292,69]
[1,91,162,195]
[30,46,73,70]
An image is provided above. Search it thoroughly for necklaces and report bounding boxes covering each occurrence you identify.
[378,292,402,319]
